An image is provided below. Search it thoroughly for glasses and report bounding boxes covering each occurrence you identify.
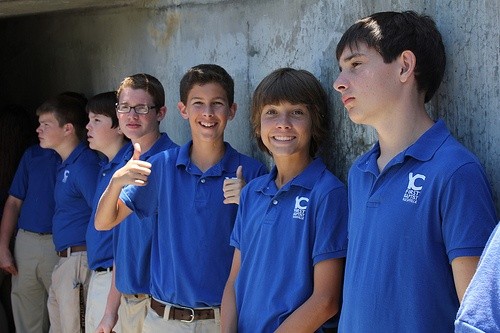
[115,103,157,114]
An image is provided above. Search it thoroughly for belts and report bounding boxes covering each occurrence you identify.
[150,297,215,323]
[94,266,113,272]
[56,244,88,258]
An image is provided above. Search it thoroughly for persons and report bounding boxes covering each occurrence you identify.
[85,91,135,333]
[94,64,268,333]
[454,220,499,332]
[333,10,497,333]
[95,73,182,333]
[1,91,87,333]
[221,68,348,333]
[35,95,103,333]
